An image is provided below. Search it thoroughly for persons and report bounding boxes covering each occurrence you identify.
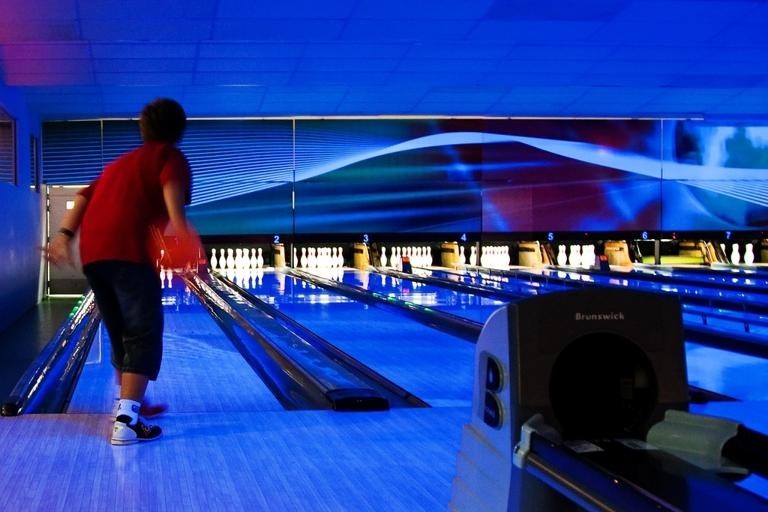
[34,96,207,444]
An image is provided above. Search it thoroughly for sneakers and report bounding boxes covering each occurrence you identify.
[110,420,163,446]
[110,396,168,425]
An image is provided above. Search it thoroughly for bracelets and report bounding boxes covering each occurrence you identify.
[58,227,74,239]
